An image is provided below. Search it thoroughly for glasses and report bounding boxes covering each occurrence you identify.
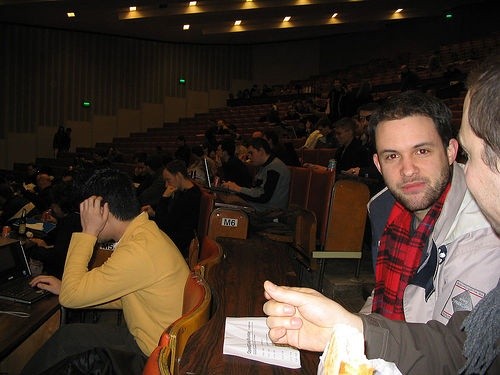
[360,115,372,121]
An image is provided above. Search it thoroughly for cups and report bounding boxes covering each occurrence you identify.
[191,170,196,179]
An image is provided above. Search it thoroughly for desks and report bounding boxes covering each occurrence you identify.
[178,236,321,375]
[0,222,108,375]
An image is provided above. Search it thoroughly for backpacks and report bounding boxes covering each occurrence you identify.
[249,204,301,233]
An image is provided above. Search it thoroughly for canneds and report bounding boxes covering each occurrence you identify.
[2,226,11,240]
[214,175,220,187]
[191,169,196,179]
[330,161,336,169]
[45,211,51,219]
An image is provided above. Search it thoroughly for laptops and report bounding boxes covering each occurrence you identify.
[203,157,237,194]
[0,239,52,304]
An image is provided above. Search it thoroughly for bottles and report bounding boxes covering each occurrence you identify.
[17,208,26,236]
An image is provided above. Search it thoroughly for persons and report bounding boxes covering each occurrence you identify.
[357,89,500,325]
[229,52,485,179]
[0,126,301,274]
[20,170,191,375]
[263,57,500,375]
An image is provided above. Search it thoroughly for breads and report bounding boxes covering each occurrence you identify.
[322,323,375,375]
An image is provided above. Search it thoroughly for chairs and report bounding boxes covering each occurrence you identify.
[0,92,500,375]
